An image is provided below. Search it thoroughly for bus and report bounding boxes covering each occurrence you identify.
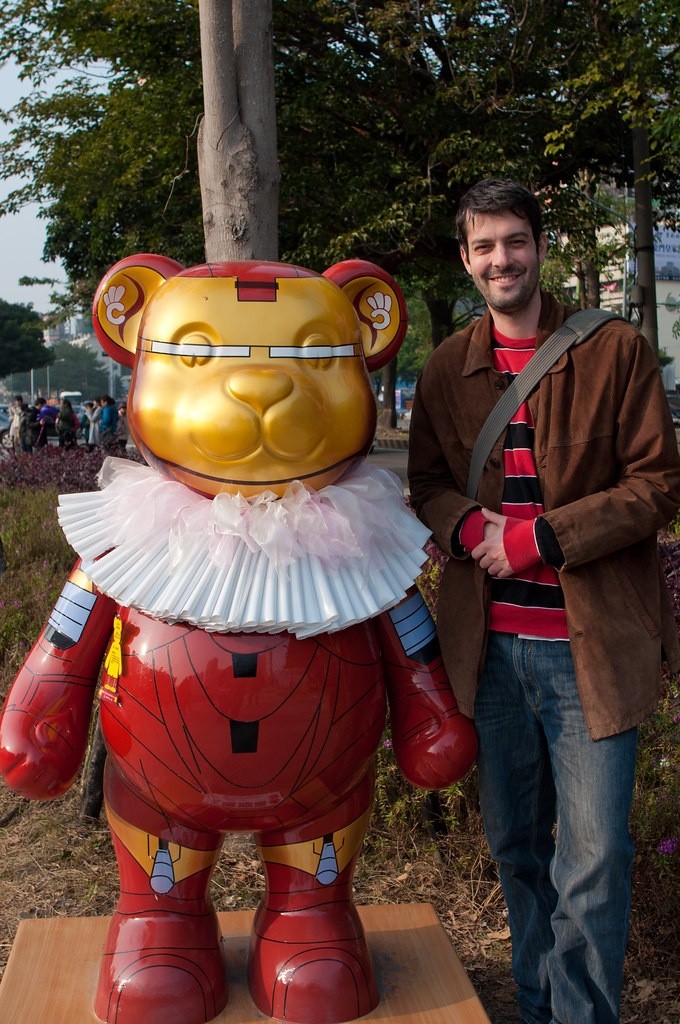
[59,391,83,414]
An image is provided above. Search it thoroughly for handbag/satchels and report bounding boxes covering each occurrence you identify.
[68,411,79,432]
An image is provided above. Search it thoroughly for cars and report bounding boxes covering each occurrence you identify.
[0,413,14,450]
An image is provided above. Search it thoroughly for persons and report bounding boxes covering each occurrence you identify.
[409,177,680,1023]
[9,395,128,452]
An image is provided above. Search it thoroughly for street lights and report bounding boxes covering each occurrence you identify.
[47,358,65,401]
